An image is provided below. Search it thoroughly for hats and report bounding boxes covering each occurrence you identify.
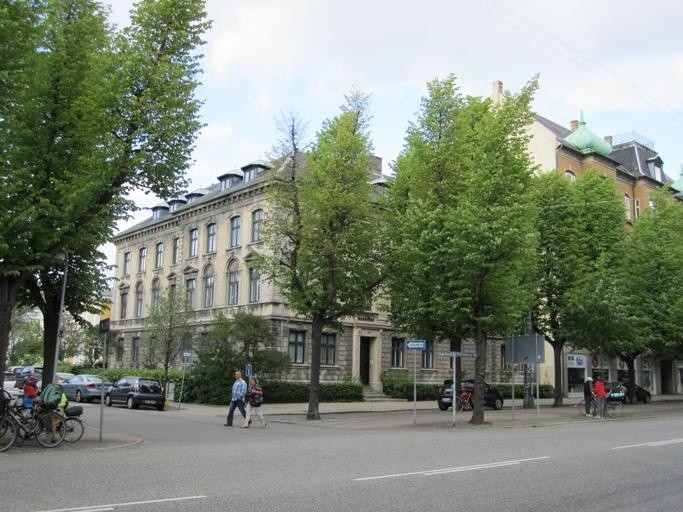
[586,376,594,382]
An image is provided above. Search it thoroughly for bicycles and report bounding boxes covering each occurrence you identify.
[579,383,625,418]
[452,390,474,414]
[0,397,84,452]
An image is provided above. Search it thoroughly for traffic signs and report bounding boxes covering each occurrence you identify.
[180,351,192,359]
[406,340,426,350]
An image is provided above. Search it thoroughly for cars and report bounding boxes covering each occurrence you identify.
[437,377,504,410]
[4,365,166,410]
[603,381,651,404]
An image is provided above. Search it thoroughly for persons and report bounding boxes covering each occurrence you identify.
[222,371,252,428]
[583,376,594,418]
[239,374,268,429]
[592,375,605,420]
[20,376,42,408]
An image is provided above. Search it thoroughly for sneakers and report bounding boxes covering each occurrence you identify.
[240,419,252,428]
[260,422,267,427]
[585,413,604,420]
[223,423,234,426]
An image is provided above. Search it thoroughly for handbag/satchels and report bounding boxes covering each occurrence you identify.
[247,395,264,407]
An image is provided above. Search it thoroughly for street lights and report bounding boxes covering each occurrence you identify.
[52,247,69,384]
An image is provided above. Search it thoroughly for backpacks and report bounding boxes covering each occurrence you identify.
[40,383,62,408]
